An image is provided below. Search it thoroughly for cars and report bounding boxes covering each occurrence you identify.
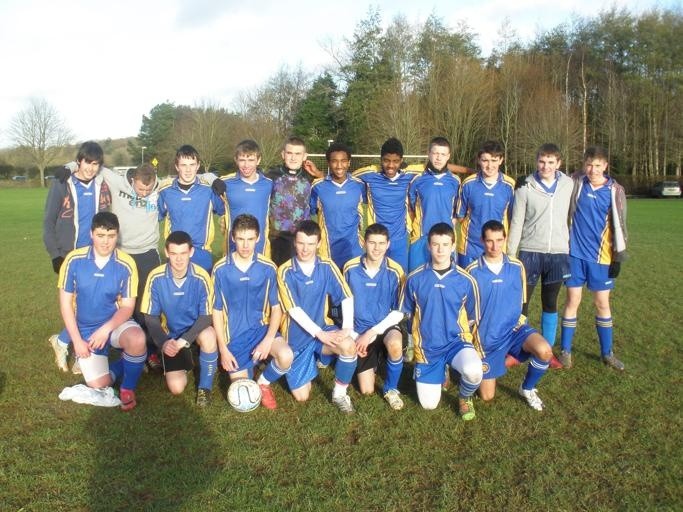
[45,176,56,180]
[12,175,26,181]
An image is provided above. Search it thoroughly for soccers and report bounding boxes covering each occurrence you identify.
[226,379,262,412]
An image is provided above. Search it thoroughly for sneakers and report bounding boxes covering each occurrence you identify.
[331,393,356,414]
[601,355,625,372]
[49,332,70,372]
[383,389,404,411]
[518,383,546,412]
[196,388,212,406]
[549,355,563,370]
[118,387,137,411]
[504,353,521,368]
[259,383,278,409]
[559,350,572,368]
[458,395,476,421]
[142,353,164,375]
[442,364,451,393]
[72,356,83,376]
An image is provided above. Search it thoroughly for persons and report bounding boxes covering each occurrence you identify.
[460,219,553,412]
[397,221,480,421]
[264,136,322,268]
[157,145,226,278]
[277,219,358,414]
[408,135,462,273]
[344,222,407,409]
[504,141,577,370]
[56,160,227,379]
[303,137,479,363]
[57,212,147,411]
[210,213,293,411]
[559,146,627,373]
[454,139,517,270]
[212,140,276,265]
[43,140,114,373]
[141,231,218,408]
[310,140,369,370]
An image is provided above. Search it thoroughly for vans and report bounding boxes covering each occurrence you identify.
[113,166,139,179]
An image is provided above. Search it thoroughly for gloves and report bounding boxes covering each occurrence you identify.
[608,262,621,278]
[52,256,65,273]
[55,166,72,184]
[515,175,527,189]
[212,177,226,195]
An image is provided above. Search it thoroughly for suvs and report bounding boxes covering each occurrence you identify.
[651,181,681,198]
